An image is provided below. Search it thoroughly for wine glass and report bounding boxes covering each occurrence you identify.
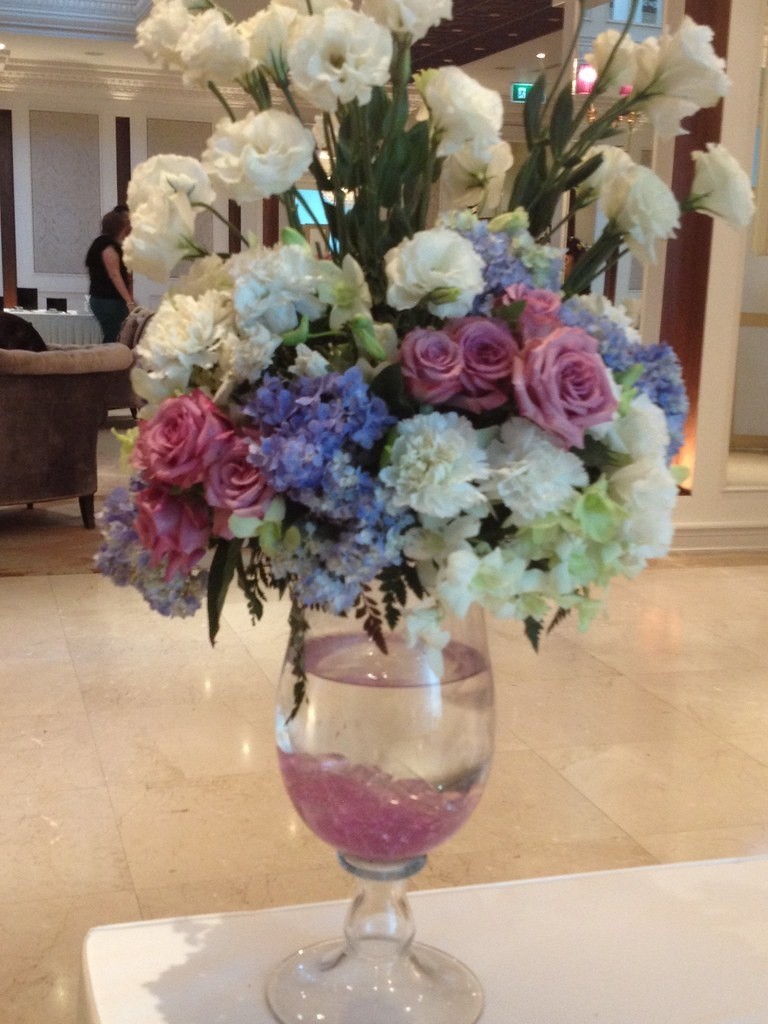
[252,582,502,1023]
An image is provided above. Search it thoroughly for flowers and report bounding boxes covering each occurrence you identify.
[81,0,761,729]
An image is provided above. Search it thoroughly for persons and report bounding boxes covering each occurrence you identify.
[114,205,133,302]
[85,211,137,343]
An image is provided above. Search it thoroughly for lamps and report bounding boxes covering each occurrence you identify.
[576,63,600,95]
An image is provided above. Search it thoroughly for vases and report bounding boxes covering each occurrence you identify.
[259,583,497,1022]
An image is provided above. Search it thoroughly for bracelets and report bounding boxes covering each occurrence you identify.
[125,302,136,306]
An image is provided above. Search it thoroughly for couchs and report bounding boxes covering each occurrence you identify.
[98,303,158,426]
[0,340,134,527]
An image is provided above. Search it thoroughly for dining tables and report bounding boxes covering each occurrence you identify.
[0,305,106,347]
